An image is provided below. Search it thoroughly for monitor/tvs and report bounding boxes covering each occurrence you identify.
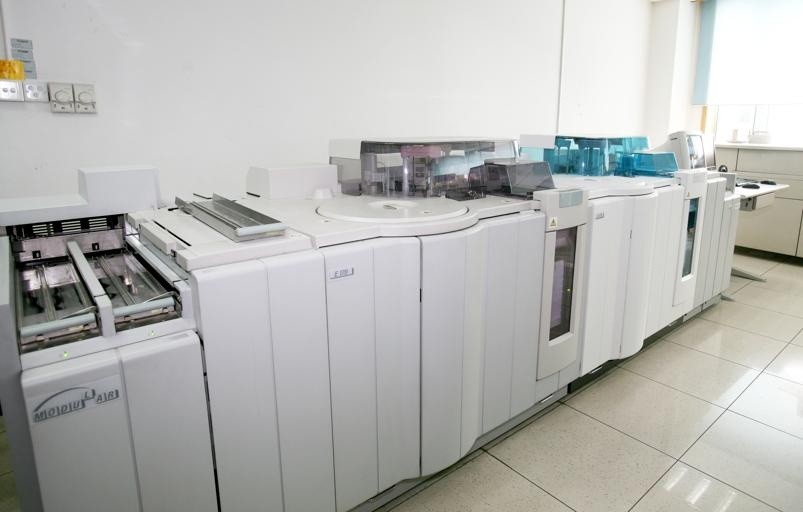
[652,130,707,170]
[706,135,716,171]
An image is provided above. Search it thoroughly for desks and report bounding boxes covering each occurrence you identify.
[731,182,789,282]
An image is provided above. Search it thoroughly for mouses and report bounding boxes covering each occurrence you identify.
[761,180,776,185]
[743,183,760,189]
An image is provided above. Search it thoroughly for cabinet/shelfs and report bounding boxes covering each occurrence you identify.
[716,148,803,257]
